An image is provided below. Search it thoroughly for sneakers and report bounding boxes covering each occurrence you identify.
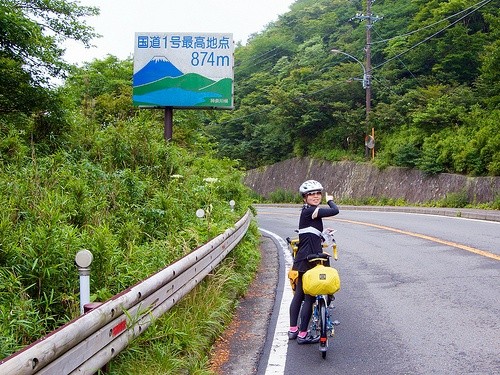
[296,332,320,344]
[288,327,311,340]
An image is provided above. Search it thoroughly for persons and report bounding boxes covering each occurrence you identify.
[288,180,340,344]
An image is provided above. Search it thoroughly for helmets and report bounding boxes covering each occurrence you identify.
[299,180,323,195]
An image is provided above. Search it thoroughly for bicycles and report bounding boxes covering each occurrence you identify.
[285,228,339,360]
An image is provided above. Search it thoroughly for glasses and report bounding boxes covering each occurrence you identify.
[306,191,322,195]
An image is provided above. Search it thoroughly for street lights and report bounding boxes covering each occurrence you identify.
[331,47,369,158]
